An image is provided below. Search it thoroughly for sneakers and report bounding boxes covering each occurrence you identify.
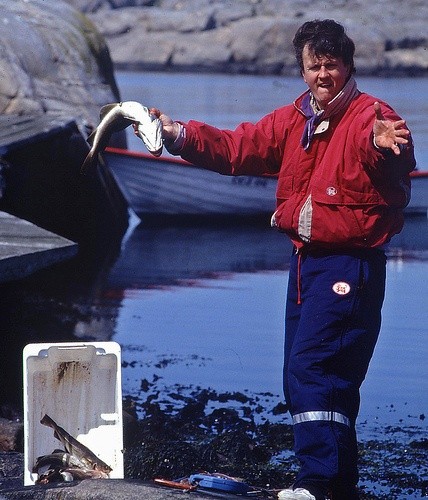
[276,485,331,500]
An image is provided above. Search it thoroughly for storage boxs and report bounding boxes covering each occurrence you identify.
[22,341,125,486]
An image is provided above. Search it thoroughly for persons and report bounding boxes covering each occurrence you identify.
[132,17,417,500]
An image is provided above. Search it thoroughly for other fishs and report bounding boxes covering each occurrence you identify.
[31,413,112,484]
[79,101,163,178]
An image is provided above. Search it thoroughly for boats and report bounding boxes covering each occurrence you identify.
[84,120,428,223]
